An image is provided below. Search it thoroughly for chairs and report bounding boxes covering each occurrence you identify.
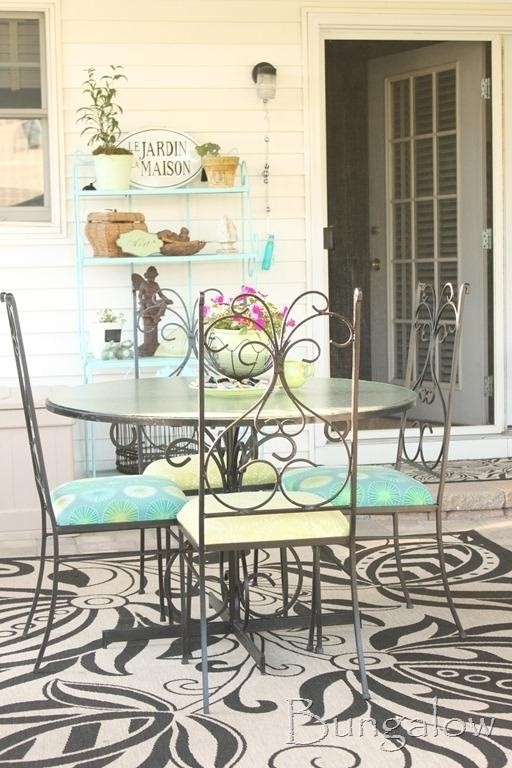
[132,286,288,623]
[180,287,371,713]
[280,276,471,651]
[1,290,189,677]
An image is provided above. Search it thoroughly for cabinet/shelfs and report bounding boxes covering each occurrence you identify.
[73,160,257,476]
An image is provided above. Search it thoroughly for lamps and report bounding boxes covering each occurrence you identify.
[249,60,279,104]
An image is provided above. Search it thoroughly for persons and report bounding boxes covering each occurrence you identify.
[131,266,175,360]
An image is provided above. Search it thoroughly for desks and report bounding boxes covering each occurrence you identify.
[47,370,418,675]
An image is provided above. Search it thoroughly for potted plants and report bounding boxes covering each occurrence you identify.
[195,143,239,188]
[75,63,133,191]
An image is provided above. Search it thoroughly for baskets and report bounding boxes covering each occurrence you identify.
[116,422,199,475]
[86,211,148,258]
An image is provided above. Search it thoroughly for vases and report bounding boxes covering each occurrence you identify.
[191,328,281,390]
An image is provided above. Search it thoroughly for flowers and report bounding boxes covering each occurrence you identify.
[194,283,296,332]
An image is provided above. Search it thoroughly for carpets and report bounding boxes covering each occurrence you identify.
[352,456,512,489]
[0,517,512,768]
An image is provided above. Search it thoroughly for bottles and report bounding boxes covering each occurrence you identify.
[261,234,275,270]
